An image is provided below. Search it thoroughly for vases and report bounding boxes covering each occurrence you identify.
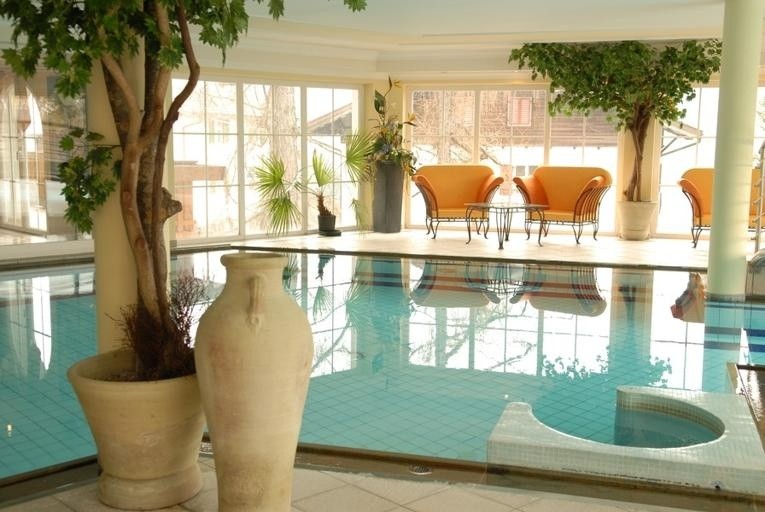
[194,253,315,511]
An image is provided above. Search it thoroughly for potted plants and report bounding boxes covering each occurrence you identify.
[363,73,419,233]
[0,0,365,512]
[507,37,723,241]
[252,127,379,237]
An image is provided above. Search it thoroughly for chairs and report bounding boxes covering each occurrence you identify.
[677,168,765,247]
[513,166,613,244]
[410,259,705,323]
[412,164,504,239]
